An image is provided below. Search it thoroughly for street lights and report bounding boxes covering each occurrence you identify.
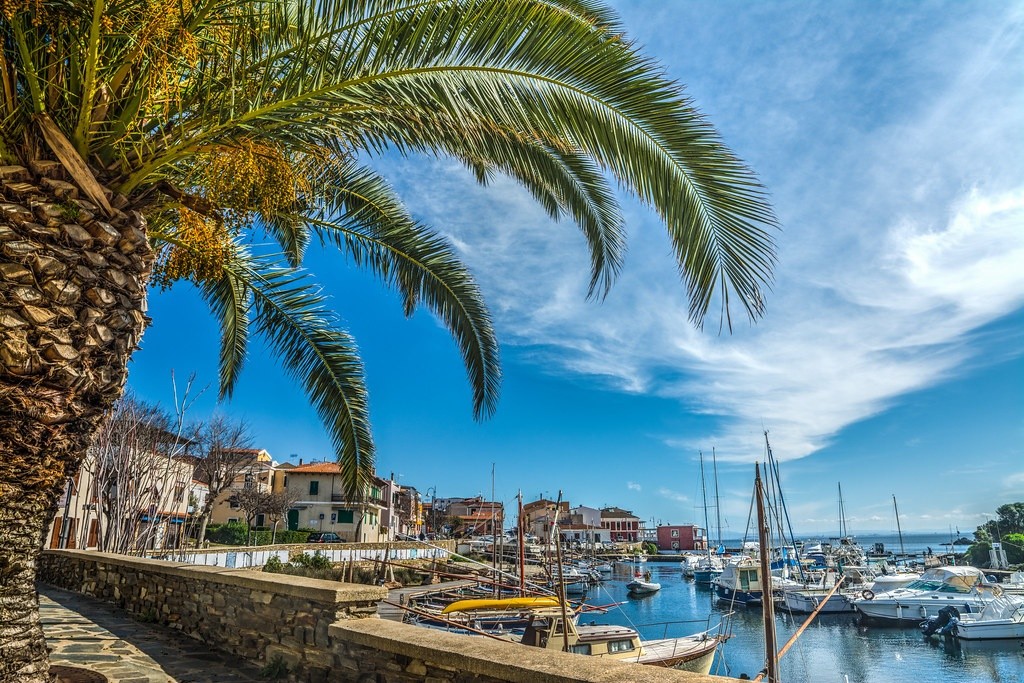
[426,487,436,531]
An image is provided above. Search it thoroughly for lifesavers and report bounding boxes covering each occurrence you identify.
[862,589,875,600]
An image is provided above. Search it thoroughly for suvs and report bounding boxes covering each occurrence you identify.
[307,531,346,543]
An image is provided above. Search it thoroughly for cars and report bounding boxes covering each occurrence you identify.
[396,530,447,542]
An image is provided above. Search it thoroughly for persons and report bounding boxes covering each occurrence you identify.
[420,531,425,540]
[635,569,651,583]
[922,546,933,559]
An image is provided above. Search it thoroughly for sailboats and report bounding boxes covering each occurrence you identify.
[401,487,734,676]
[679,428,1024,638]
